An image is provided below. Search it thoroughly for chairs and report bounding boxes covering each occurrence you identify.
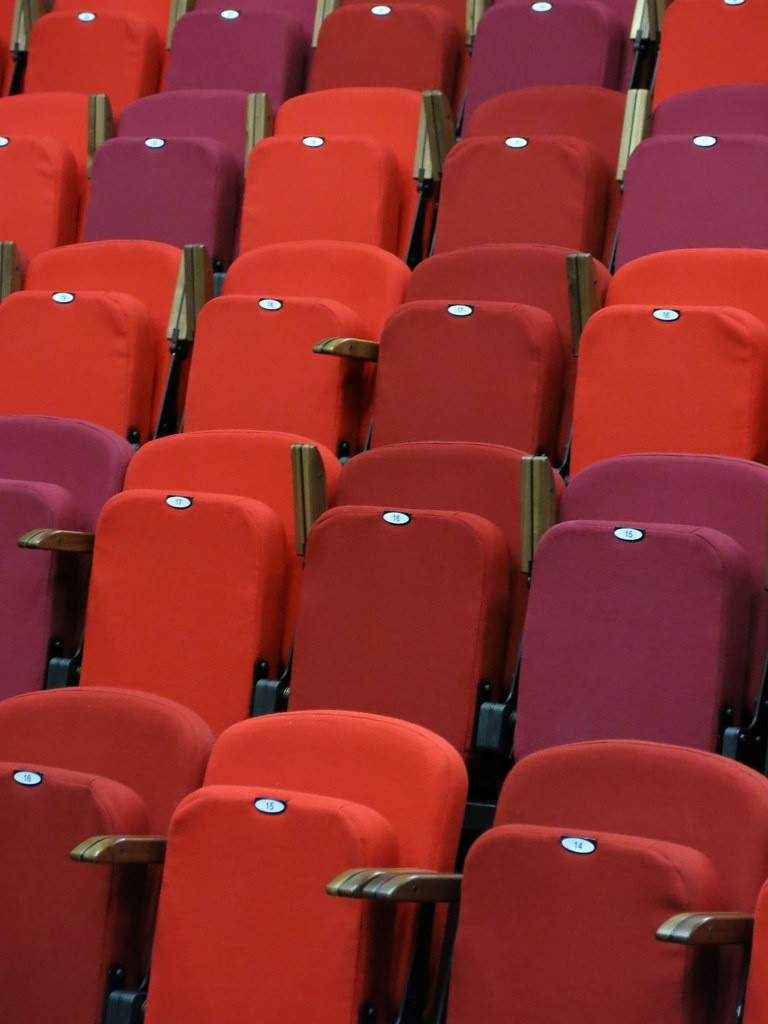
[0,0,767,1024]
